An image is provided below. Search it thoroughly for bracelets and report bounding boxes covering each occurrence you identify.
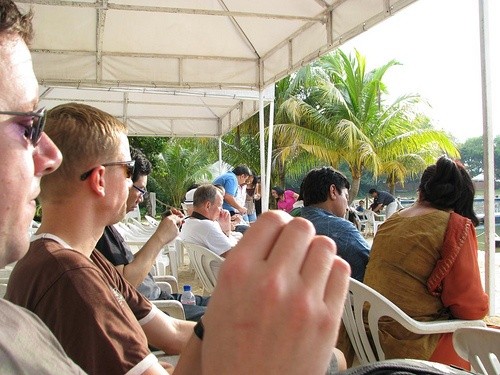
[194,319,204,339]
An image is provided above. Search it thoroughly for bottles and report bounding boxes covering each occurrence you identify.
[181,285,196,305]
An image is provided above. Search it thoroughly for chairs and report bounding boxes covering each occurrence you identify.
[113,214,226,367]
[293,200,500,375]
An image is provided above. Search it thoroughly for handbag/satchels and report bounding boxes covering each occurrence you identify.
[245,201,255,216]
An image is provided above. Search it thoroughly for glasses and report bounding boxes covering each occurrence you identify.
[132,184,147,198]
[80,159,135,181]
[0,106,47,148]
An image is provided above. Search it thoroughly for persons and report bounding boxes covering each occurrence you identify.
[95,144,212,321]
[181,164,299,259]
[4,102,347,375]
[360,157,500,370]
[0,0,351,375]
[369,189,397,219]
[299,167,370,284]
[348,200,382,231]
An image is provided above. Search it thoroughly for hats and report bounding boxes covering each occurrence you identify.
[184,188,196,205]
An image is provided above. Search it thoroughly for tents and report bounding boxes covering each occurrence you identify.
[9,0,496,317]
[36,82,275,216]
